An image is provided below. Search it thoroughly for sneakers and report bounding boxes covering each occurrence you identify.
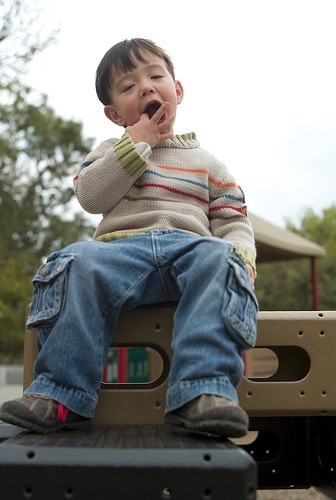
[164,394,248,438]
[0,393,92,435]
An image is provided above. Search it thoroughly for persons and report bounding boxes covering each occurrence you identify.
[0,38,260,438]
